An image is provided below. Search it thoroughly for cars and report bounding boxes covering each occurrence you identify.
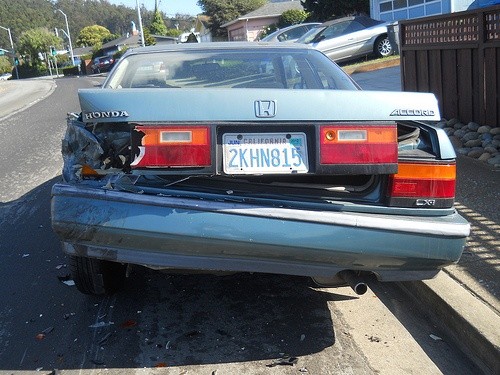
[291,15,396,78]
[49,39,471,298]
[250,21,336,44]
[90,55,116,74]
[128,60,170,88]
[0,72,12,80]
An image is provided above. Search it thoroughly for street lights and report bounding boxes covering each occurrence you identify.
[54,9,75,67]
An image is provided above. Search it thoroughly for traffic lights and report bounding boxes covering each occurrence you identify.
[51,46,56,56]
[15,57,19,64]
